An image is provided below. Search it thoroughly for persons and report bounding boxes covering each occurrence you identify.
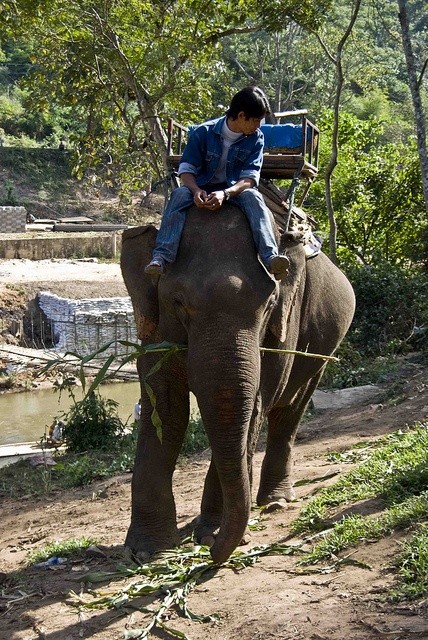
[144,86,290,280]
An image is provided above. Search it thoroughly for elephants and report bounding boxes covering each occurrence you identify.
[119,179,356,567]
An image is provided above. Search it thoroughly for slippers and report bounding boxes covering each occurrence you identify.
[145,258,165,274]
[267,255,290,280]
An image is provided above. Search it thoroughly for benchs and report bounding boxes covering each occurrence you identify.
[167,108,319,220]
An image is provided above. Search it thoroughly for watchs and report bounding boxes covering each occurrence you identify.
[223,189,230,200]
